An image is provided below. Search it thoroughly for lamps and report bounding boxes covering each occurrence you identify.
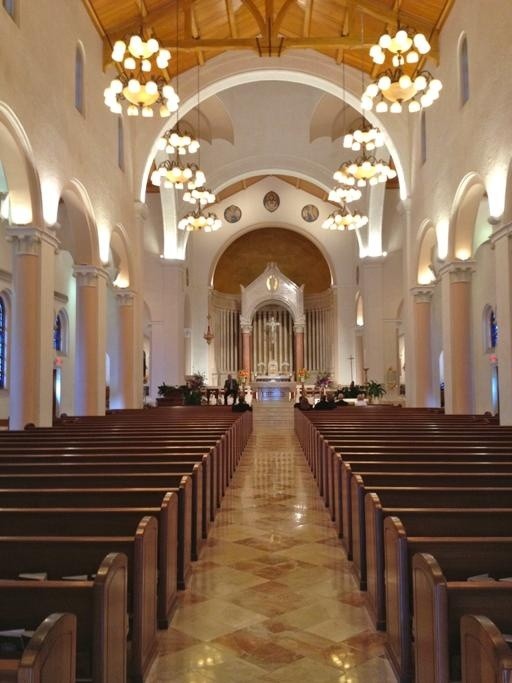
[362,16,443,117]
[98,24,181,119]
[151,130,222,233]
[324,126,399,232]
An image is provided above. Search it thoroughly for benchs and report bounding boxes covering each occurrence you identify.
[1,406,254,589]
[2,515,161,681]
[1,552,130,681]
[296,405,511,587]
[0,492,180,628]
[412,554,512,680]
[363,492,512,682]
[1,612,77,680]
[461,614,512,682]
[382,514,512,682]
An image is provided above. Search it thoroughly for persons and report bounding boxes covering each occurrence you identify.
[300,398,312,410]
[233,397,249,412]
[224,374,238,406]
[355,394,367,406]
[336,394,349,406]
[323,395,337,409]
[315,395,327,408]
